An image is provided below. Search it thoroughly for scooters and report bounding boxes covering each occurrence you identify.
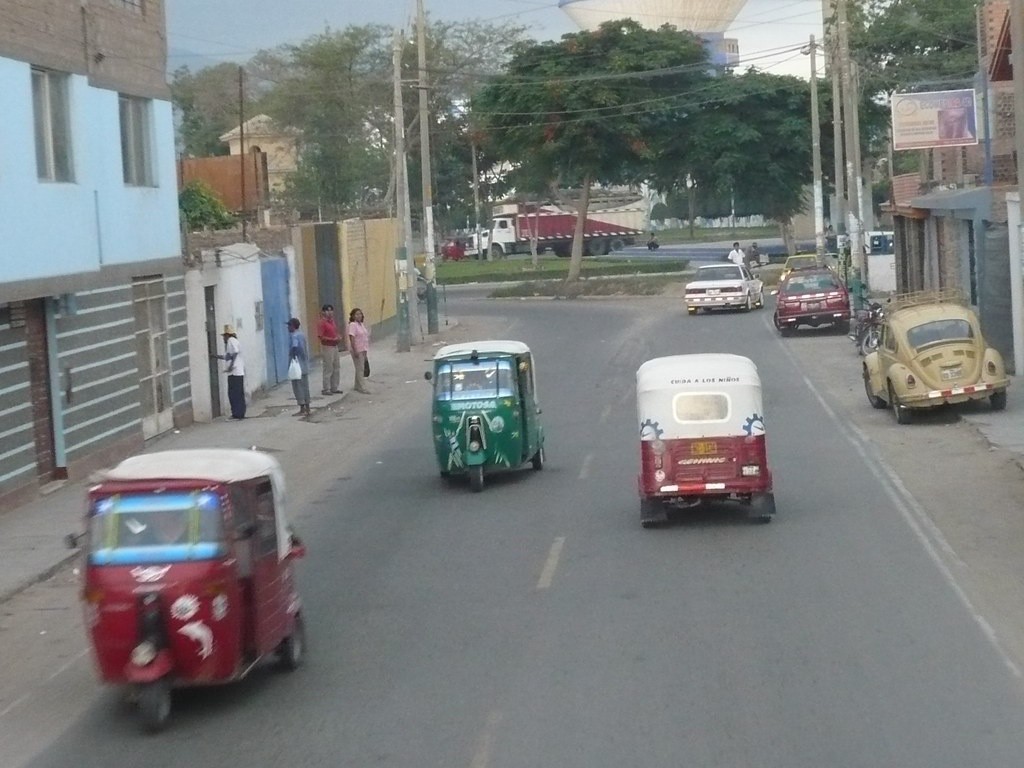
[648,241,657,253]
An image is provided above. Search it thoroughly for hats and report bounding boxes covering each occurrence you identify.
[220,324,237,335]
[285,318,300,327]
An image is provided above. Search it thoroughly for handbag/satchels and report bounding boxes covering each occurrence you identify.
[364,355,370,377]
[287,356,302,380]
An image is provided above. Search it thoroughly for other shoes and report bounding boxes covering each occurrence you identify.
[225,416,239,421]
[330,390,343,393]
[292,412,308,416]
[323,391,333,395]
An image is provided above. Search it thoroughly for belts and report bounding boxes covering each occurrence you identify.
[321,342,337,346]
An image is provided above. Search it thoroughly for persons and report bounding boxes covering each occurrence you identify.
[728,242,746,264]
[138,510,190,545]
[825,225,837,246]
[284,318,311,416]
[318,305,344,395]
[751,242,758,259]
[841,227,849,236]
[347,309,369,394]
[212,325,246,421]
[647,233,659,251]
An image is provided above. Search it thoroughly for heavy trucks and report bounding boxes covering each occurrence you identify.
[464,207,646,261]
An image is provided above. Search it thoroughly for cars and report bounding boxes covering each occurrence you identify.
[864,303,1012,416]
[772,265,854,339]
[686,264,767,314]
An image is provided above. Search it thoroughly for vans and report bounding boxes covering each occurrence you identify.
[780,253,839,285]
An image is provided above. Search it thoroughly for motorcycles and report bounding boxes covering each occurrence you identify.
[423,341,545,492]
[441,236,466,262]
[632,353,782,528]
[66,447,309,729]
